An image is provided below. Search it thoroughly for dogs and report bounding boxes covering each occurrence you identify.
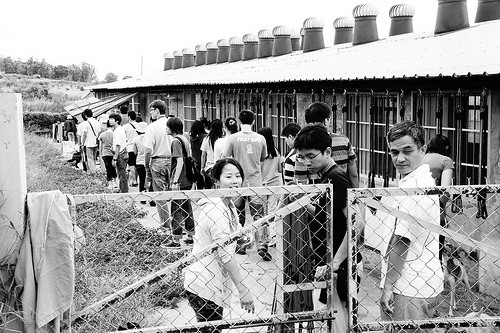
[67,151,82,168]
[442,243,478,316]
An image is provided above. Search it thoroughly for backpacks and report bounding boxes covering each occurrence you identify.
[175,137,204,189]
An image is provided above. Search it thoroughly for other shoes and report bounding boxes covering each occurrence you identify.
[165,242,180,248]
[141,201,146,204]
[268,237,276,246]
[131,182,138,187]
[235,245,246,255]
[150,201,156,206]
[258,248,272,261]
[187,239,194,246]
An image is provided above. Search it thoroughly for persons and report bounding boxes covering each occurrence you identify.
[64,99,281,260]
[378,120,454,333]
[185,157,255,333]
[281,103,362,333]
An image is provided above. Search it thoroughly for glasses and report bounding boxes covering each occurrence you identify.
[297,151,321,162]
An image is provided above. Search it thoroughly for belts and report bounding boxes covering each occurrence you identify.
[153,157,171,159]
